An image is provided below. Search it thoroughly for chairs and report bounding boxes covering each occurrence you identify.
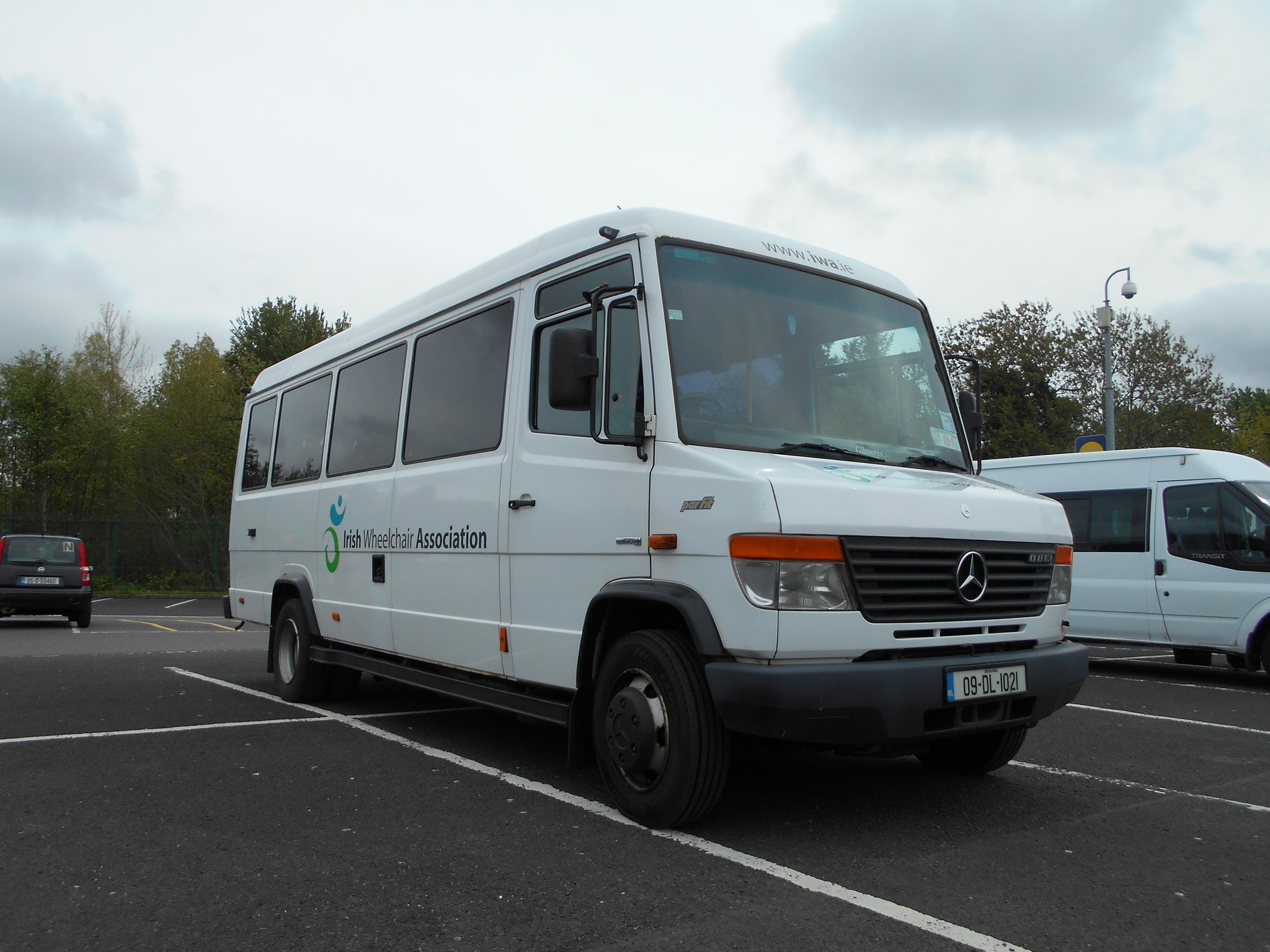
[1168,506,1252,551]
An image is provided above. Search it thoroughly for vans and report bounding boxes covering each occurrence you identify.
[972,446,1270,682]
[220,203,1091,831]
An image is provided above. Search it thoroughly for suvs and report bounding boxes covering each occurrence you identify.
[0,533,94,628]
